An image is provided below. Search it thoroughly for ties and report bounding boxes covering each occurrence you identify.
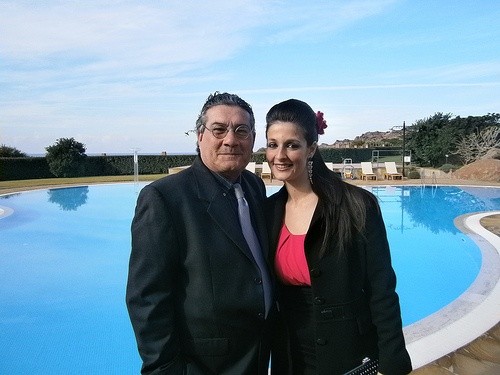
[231,183,276,321]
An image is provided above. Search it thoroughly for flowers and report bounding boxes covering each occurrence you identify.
[317,111,328,135]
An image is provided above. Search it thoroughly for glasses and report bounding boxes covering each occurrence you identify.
[202,123,255,140]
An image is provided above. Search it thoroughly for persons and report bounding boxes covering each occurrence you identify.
[126,92,275,375]
[267,99,412,375]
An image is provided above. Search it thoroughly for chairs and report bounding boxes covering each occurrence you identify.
[244,161,258,176]
[259,162,273,183]
[324,162,333,171]
[384,161,404,181]
[360,162,378,182]
